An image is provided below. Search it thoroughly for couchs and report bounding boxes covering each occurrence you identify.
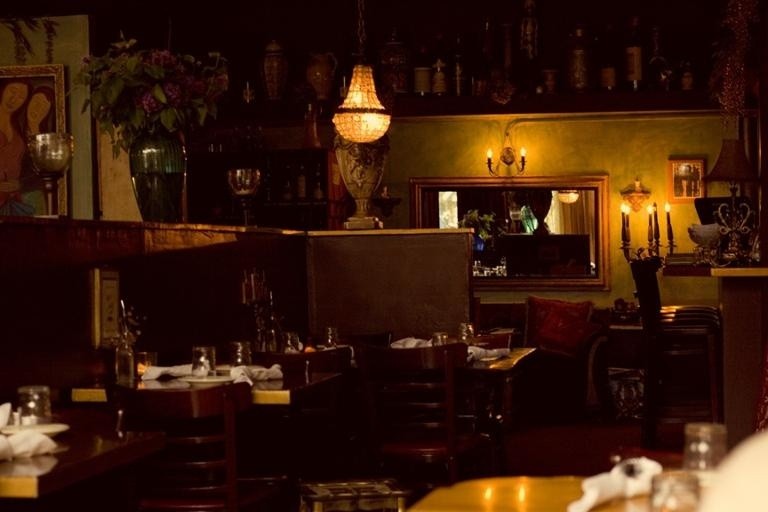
[481,303,604,423]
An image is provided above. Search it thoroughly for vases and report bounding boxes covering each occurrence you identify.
[129,134,183,223]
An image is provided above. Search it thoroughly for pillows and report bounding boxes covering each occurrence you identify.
[523,293,593,358]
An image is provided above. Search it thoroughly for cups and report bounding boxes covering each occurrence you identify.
[136,352,159,378]
[189,343,216,378]
[17,384,51,425]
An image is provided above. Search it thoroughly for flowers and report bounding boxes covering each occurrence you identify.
[65,30,228,159]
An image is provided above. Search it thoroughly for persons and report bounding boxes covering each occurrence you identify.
[674,164,701,196]
[0,79,28,209]
[24,85,54,159]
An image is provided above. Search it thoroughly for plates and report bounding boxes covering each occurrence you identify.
[2,423,69,440]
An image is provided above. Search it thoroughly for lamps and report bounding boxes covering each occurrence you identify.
[619,203,677,271]
[330,0,393,145]
[486,147,527,176]
[558,189,579,204]
[704,137,758,260]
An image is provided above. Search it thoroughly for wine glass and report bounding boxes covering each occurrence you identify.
[27,131,74,219]
[229,167,260,227]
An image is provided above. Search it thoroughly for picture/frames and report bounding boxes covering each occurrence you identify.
[666,159,705,203]
[0,64,69,218]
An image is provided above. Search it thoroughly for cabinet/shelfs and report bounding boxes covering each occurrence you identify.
[710,268,768,453]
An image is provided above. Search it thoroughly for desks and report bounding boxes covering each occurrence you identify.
[592,319,644,415]
[458,345,537,435]
[0,411,168,512]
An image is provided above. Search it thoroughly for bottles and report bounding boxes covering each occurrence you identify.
[644,420,727,511]
[241,36,352,103]
[378,15,693,96]
[238,267,288,350]
[115,330,136,383]
[280,162,325,198]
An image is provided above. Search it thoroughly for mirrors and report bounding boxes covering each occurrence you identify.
[410,177,611,293]
